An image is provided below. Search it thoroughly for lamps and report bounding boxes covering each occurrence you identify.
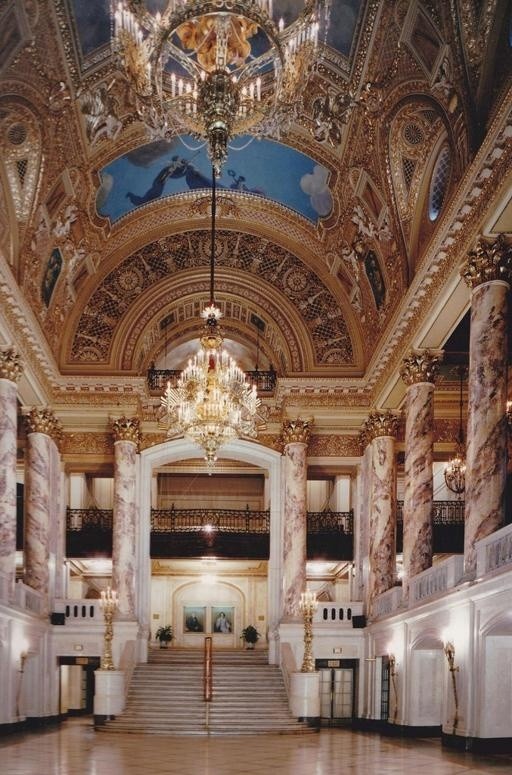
[16,653,29,723]
[445,642,460,735]
[389,655,398,728]
[444,366,467,502]
[154,69,261,479]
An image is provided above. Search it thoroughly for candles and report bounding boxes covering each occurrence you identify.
[98,586,118,609]
[298,587,318,612]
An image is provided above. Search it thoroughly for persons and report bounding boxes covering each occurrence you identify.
[215,612,231,633]
[187,613,201,632]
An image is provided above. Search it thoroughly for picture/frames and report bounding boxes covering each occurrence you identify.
[183,606,207,635]
[210,606,236,637]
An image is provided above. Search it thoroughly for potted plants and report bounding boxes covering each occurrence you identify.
[155,625,176,649]
[239,625,261,649]
